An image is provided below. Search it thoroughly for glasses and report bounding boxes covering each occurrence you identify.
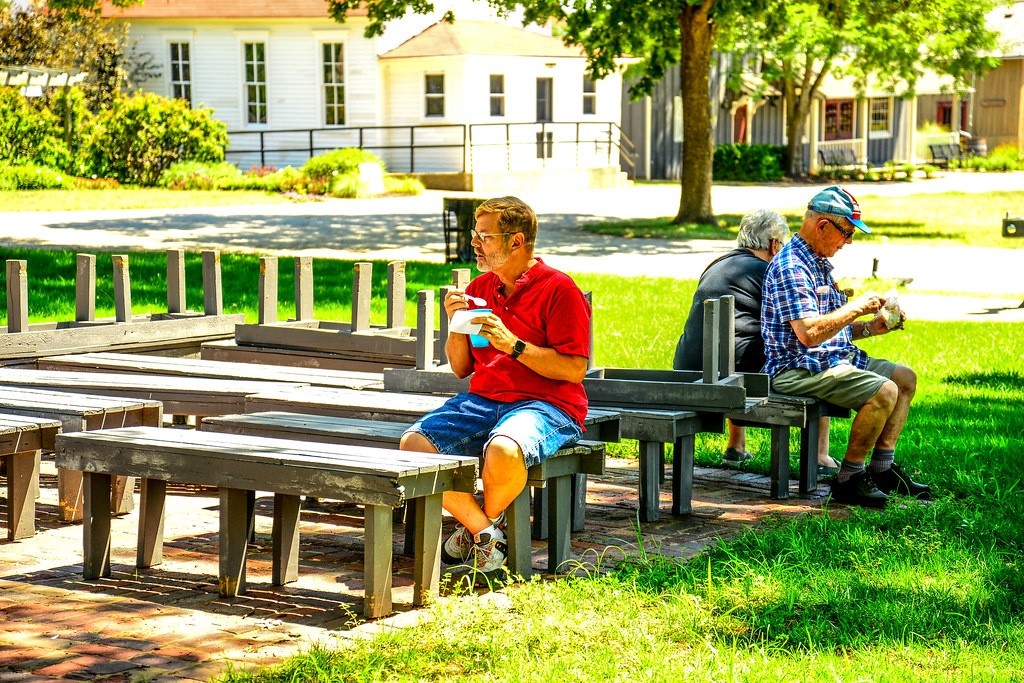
[817,217,856,240]
[470,229,523,242]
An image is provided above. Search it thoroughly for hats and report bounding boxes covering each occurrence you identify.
[807,185,871,234]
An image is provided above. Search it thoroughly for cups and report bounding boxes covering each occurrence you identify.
[880,297,902,331]
[468,309,492,348]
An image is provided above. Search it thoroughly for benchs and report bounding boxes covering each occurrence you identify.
[930,144,970,170]
[819,148,862,165]
[0,249,852,618]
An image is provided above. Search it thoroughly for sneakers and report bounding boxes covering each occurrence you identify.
[441,502,508,566]
[865,462,932,500]
[830,470,895,509]
[462,526,508,588]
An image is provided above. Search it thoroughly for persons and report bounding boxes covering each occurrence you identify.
[761,186,930,509]
[400,197,593,584]
[672,208,842,477]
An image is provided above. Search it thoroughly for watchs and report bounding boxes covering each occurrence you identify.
[510,339,526,359]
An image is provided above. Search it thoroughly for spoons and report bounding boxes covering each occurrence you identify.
[463,294,487,306]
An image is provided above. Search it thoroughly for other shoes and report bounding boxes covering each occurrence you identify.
[816,456,842,481]
[722,447,754,466]
